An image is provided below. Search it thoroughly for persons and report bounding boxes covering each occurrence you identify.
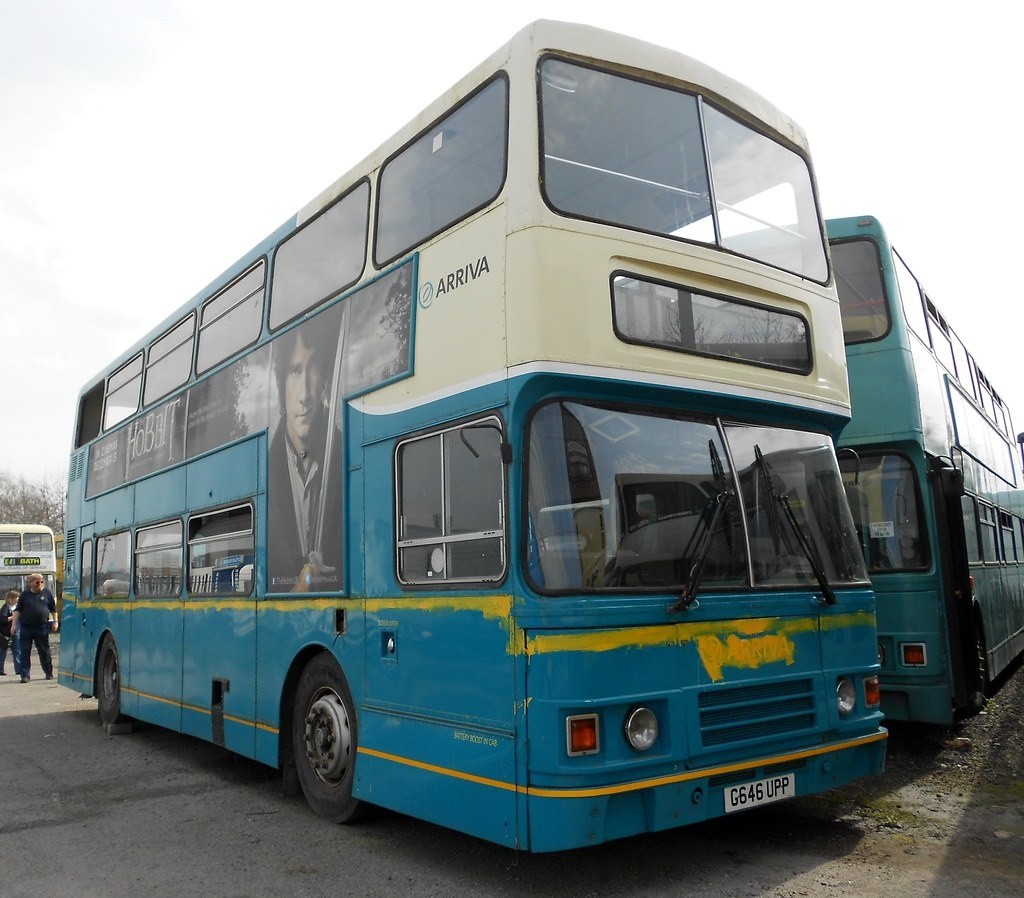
[266,315,343,595]
[0,591,21,676]
[10,573,58,683]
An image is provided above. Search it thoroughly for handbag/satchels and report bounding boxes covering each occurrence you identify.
[0,632,13,649]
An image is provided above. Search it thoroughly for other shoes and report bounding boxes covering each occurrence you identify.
[0,669,7,676]
[45,672,54,680]
[20,675,32,683]
[14,670,21,675]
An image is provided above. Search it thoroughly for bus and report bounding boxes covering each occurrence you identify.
[0,523,57,631]
[819,193,1024,741]
[56,10,891,863]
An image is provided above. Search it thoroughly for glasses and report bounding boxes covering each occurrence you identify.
[33,580,45,584]
